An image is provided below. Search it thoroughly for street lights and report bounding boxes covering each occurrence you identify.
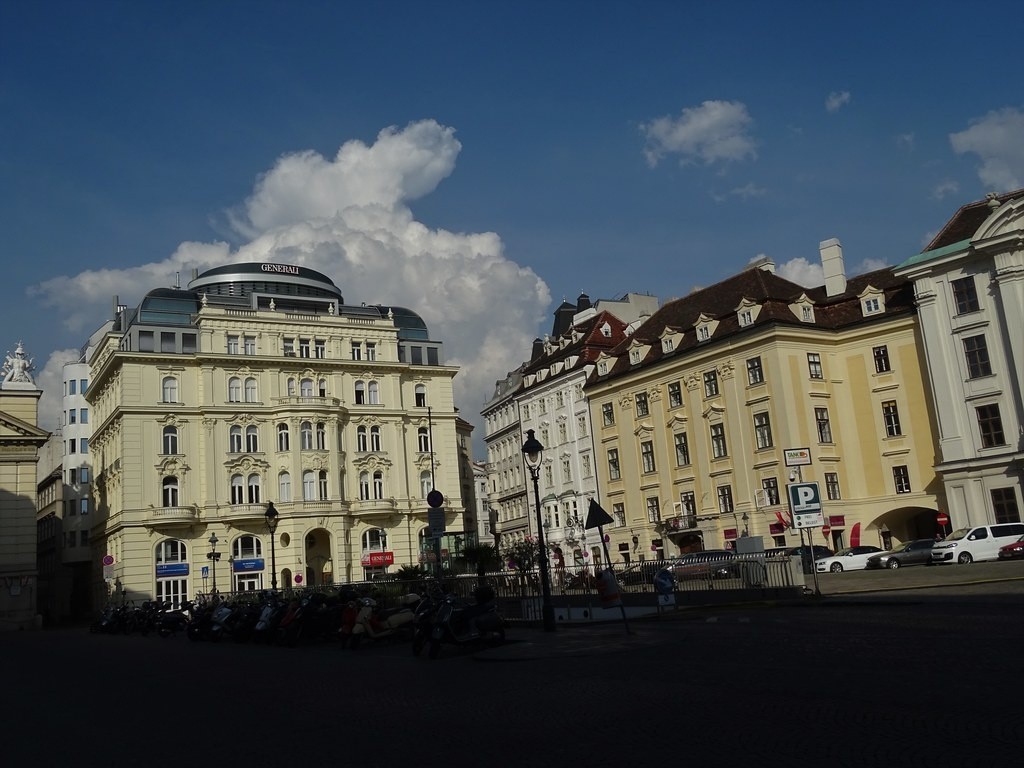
[522,429,559,633]
[542,518,553,591]
[742,512,749,536]
[378,528,387,574]
[209,532,219,594]
[265,503,279,589]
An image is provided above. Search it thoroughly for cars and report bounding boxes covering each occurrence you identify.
[811,545,891,573]
[998,534,1024,559]
[615,564,665,585]
[868,538,936,569]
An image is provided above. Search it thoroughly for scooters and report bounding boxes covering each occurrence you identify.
[186,586,422,651]
[412,580,510,659]
[90,599,197,638]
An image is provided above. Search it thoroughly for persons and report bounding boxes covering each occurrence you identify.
[7,348,30,382]
[935,534,942,539]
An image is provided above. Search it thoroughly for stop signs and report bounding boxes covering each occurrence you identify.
[936,514,948,525]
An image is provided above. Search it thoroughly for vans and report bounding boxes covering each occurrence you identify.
[931,521,1024,563]
[660,550,736,581]
[775,545,833,573]
